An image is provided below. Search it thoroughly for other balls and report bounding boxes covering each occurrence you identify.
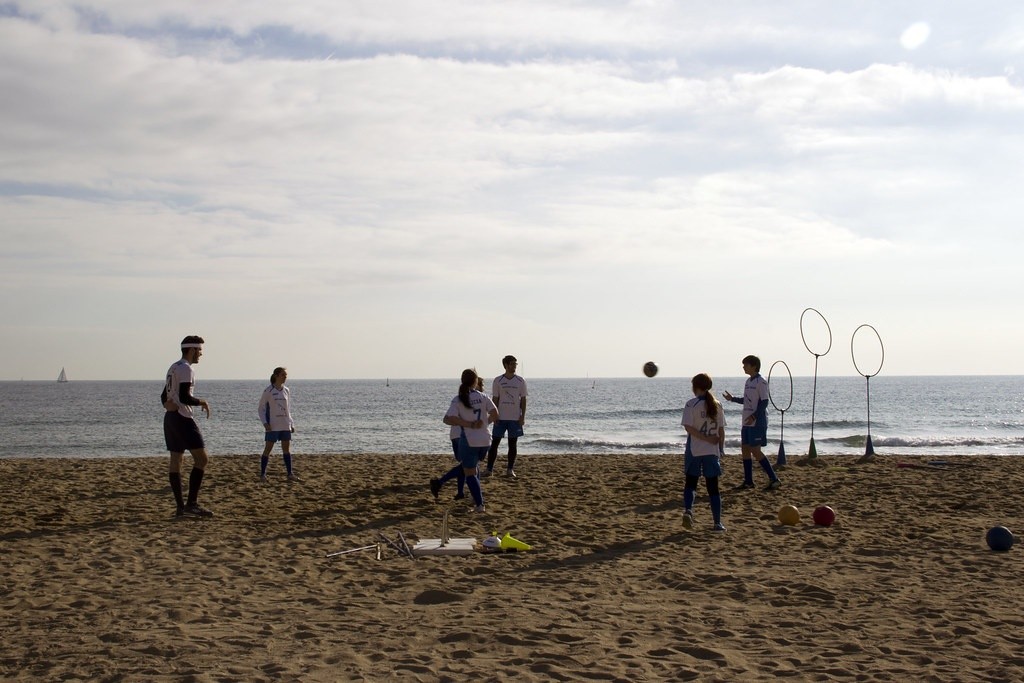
[985,525,1014,551]
[779,505,800,524]
[813,505,835,526]
[643,361,658,378]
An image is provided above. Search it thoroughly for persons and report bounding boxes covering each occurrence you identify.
[723,356,782,492]
[430,368,500,513]
[258,367,306,483]
[483,355,528,477]
[161,335,214,519]
[680,373,727,531]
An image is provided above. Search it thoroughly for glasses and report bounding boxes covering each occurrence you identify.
[509,362,518,366]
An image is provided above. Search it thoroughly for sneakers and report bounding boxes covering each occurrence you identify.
[713,523,727,532]
[682,511,694,530]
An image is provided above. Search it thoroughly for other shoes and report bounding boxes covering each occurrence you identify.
[732,481,757,490]
[430,479,440,498]
[507,469,516,478]
[763,479,782,492]
[474,505,485,513]
[288,475,305,483]
[480,469,493,477]
[176,510,183,518]
[185,504,214,517]
